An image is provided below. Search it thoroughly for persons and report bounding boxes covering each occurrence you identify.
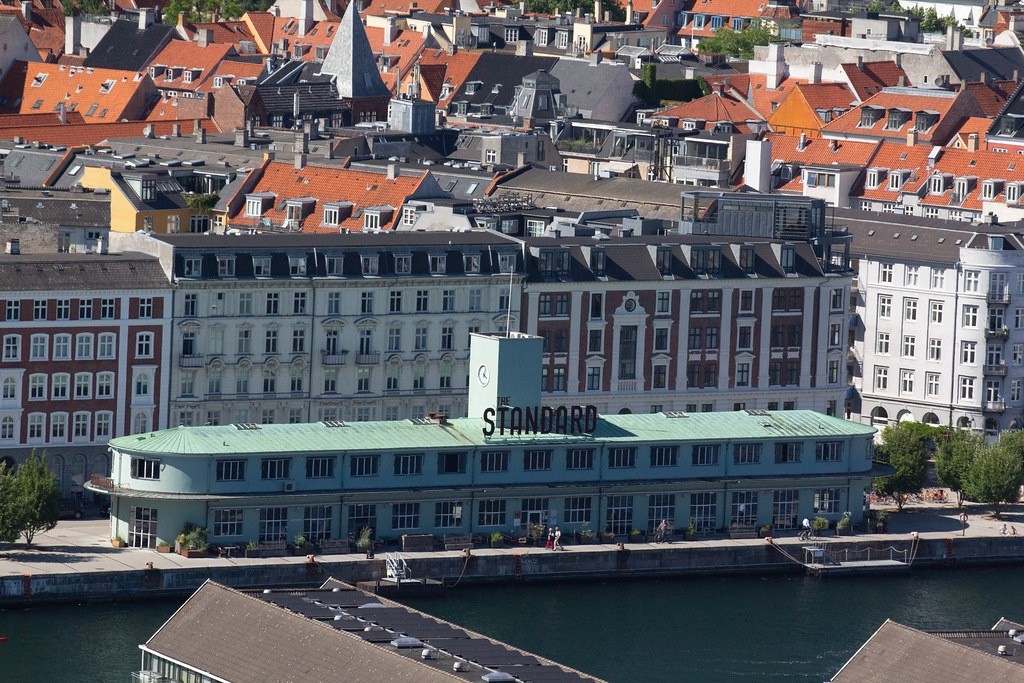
[657,519,667,543]
[552,527,563,551]
[545,528,554,550]
[999,524,1007,536]
[802,516,810,540]
[1011,526,1017,535]
